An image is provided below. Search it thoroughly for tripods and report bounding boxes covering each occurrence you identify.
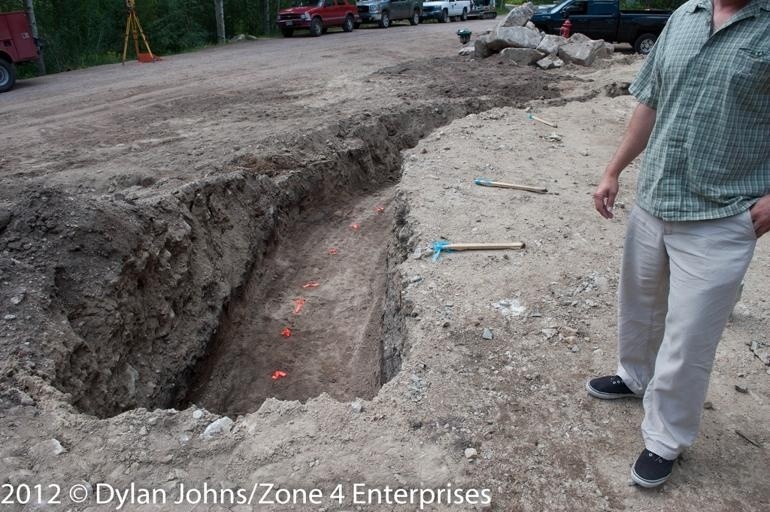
[122,9,154,65]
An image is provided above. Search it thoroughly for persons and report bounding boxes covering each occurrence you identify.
[585,0,770,488]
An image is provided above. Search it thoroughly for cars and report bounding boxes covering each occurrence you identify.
[276,0,363,37]
[420,0,472,22]
[356,0,423,27]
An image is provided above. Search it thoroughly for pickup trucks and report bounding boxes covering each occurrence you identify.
[529,0,673,56]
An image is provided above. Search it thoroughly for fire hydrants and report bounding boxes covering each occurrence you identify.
[560,19,573,38]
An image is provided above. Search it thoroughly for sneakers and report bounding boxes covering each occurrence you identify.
[585,375,640,399]
[631,447,673,488]
